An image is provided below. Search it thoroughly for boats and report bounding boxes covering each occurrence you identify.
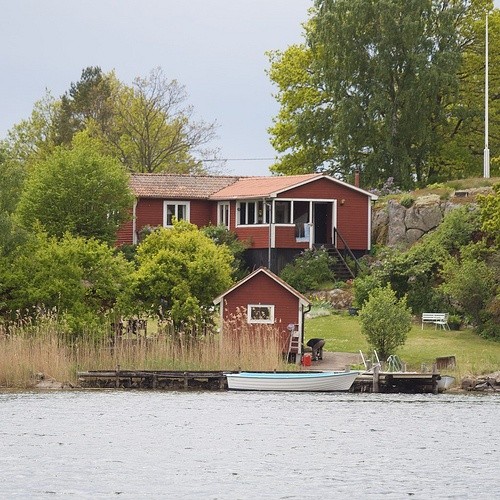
[224,370,360,392]
[414,375,457,392]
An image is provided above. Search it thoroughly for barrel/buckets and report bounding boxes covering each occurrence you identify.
[302,355,311,366]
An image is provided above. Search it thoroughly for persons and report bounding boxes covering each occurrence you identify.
[306,338,325,361]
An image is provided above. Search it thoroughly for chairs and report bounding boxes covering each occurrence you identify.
[433,312,451,331]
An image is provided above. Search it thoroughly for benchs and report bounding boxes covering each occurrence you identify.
[421,312,446,331]
